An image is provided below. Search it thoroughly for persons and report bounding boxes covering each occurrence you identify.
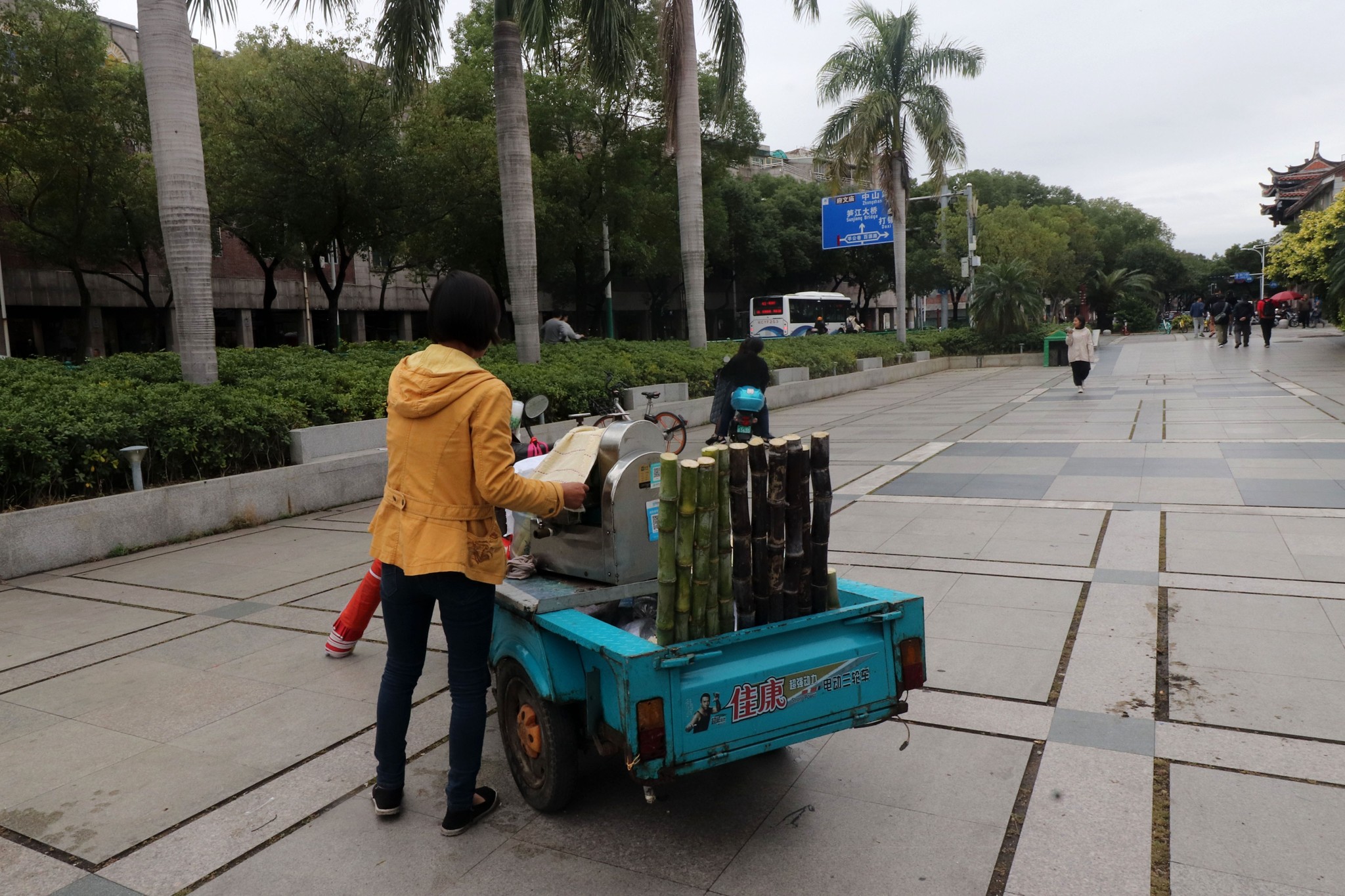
[1296,293,1327,328]
[705,340,776,446]
[714,338,774,447]
[1065,314,1094,393]
[539,308,564,344]
[845,311,865,334]
[560,310,585,342]
[814,316,828,335]
[1189,287,1281,349]
[369,269,589,838]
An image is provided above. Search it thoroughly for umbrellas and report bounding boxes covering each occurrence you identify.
[1270,290,1304,302]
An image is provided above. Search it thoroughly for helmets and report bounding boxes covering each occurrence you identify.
[817,316,822,320]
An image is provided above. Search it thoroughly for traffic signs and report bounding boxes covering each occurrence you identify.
[821,190,894,249]
[1235,272,1249,279]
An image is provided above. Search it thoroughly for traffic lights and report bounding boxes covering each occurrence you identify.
[1208,275,1224,281]
[1207,284,1215,295]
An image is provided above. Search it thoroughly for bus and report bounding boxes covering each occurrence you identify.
[750,291,852,338]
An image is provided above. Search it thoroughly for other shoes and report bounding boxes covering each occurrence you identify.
[1209,332,1215,337]
[1235,342,1241,348]
[763,437,771,446]
[1199,332,1205,337]
[705,434,726,445]
[1077,380,1085,393]
[370,782,400,815]
[1244,345,1249,346]
[1218,344,1223,347]
[439,786,498,836]
[1311,325,1316,328]
[1194,334,1198,338]
[1322,323,1325,327]
[1265,345,1269,347]
[1229,333,1232,336]
[1302,327,1305,328]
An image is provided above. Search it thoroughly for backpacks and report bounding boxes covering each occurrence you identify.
[1262,298,1274,316]
[846,317,855,334]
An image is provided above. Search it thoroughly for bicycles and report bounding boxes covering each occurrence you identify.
[588,371,688,455]
[1158,311,1171,334]
[1203,318,1210,332]
[1172,312,1188,333]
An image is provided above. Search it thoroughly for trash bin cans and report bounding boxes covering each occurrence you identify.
[1043,329,1068,366]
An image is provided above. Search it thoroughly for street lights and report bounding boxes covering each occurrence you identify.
[1240,248,1264,299]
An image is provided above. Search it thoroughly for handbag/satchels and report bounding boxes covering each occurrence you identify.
[1214,312,1226,323]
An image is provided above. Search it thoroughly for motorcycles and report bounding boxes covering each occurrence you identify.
[1274,303,1299,327]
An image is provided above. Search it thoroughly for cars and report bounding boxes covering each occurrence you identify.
[1160,311,1210,322]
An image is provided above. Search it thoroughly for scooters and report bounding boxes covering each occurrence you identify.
[490,394,928,815]
[722,356,765,444]
[811,322,829,336]
